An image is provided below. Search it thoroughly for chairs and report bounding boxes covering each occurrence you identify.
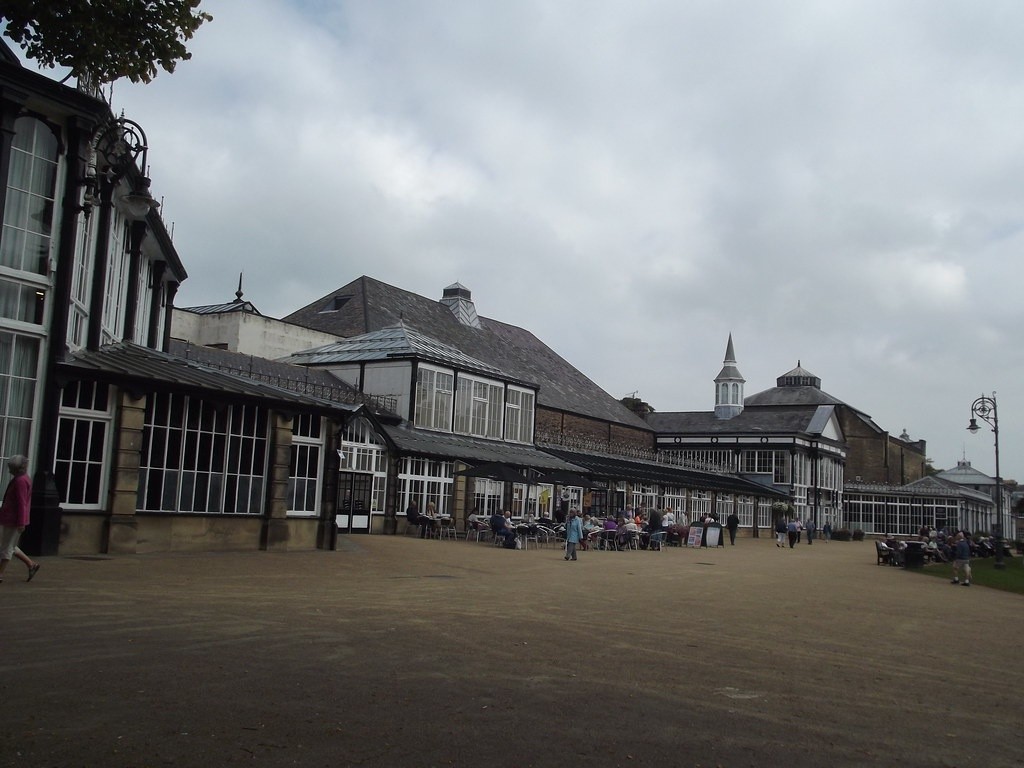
[874,539,996,569]
[405,513,690,551]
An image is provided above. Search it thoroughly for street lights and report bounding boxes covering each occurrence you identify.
[965,390,1006,571]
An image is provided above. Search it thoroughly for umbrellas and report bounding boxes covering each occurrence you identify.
[535,469,604,513]
[454,462,539,513]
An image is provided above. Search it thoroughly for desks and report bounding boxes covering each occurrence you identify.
[424,518,446,540]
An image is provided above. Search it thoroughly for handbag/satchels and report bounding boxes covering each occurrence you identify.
[514,538,521,550]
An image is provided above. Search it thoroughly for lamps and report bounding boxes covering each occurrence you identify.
[76,109,157,222]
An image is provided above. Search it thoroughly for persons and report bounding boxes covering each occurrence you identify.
[524,511,535,523]
[468,507,519,549]
[561,503,719,561]
[543,512,549,519]
[408,501,438,539]
[878,527,1011,587]
[727,511,739,545]
[776,514,831,548]
[0,455,40,583]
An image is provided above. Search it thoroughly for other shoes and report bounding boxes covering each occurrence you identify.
[782,546,784,547]
[961,583,970,586]
[26,562,40,582]
[951,579,959,583]
[571,559,577,560]
[777,544,780,548]
[565,556,569,560]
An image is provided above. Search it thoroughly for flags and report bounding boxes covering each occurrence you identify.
[540,491,549,504]
[584,494,591,506]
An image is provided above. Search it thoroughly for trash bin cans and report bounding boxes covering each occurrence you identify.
[903,539,926,569]
[20,469,63,556]
